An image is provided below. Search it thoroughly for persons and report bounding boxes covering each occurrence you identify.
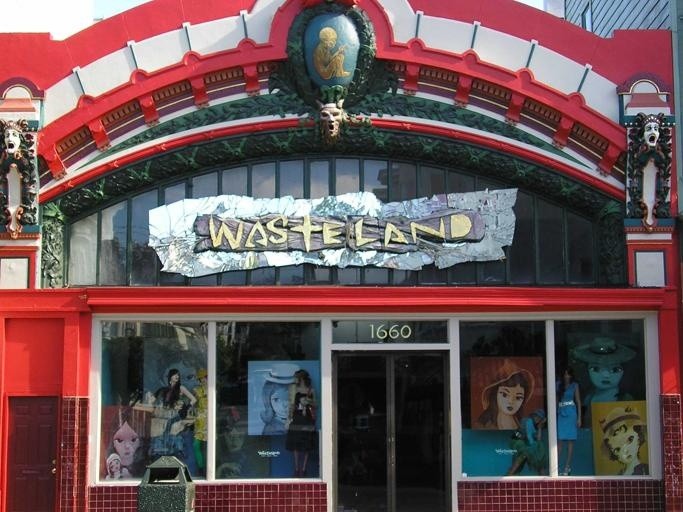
[257,363,301,435]
[472,360,535,431]
[212,406,264,479]
[598,405,648,476]
[0,119,29,154]
[311,22,352,83]
[506,408,547,476]
[286,369,319,478]
[554,366,582,477]
[106,453,133,481]
[150,368,198,458]
[570,336,636,408]
[106,406,146,480]
[639,112,666,150]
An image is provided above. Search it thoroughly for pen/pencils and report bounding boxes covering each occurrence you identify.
[137,455,196,512]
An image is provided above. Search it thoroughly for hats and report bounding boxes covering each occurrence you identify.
[264,364,300,384]
[572,337,637,364]
[106,453,121,476]
[532,409,545,420]
[482,363,536,410]
[195,369,207,380]
[598,405,646,441]
[310,404,316,421]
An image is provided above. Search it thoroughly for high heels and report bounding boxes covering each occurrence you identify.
[295,468,306,477]
[562,466,571,475]
